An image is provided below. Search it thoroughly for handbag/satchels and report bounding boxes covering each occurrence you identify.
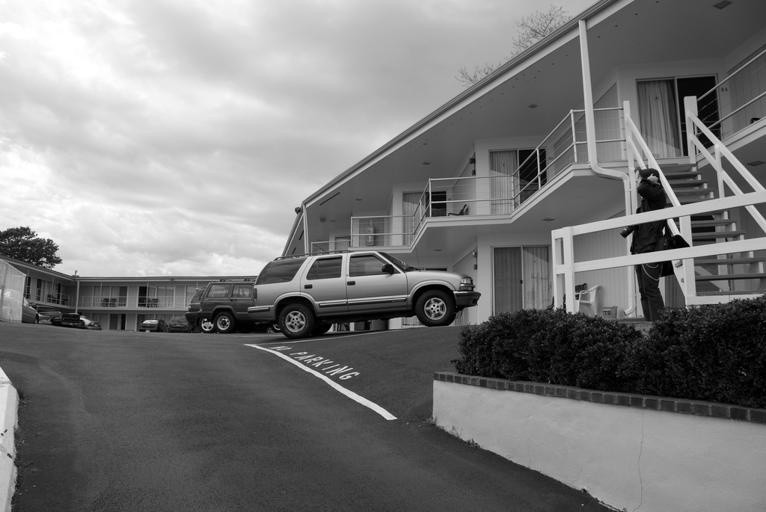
[664,234,689,249]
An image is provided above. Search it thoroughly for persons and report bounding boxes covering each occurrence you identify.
[618,167,673,326]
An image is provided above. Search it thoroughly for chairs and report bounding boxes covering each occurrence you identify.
[577,283,602,318]
[446,203,466,216]
[45,293,159,309]
[552,283,587,312]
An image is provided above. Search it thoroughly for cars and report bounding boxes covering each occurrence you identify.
[0,287,39,325]
[139,319,164,332]
[163,317,188,333]
[38,310,101,331]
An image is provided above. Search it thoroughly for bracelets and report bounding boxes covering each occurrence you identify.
[624,227,629,235]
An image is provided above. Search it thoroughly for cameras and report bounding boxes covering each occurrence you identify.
[621,227,634,239]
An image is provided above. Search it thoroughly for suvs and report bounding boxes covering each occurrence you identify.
[184,287,214,333]
[200,279,281,333]
[246,249,480,339]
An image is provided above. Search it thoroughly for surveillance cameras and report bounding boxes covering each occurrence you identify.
[295,205,301,213]
